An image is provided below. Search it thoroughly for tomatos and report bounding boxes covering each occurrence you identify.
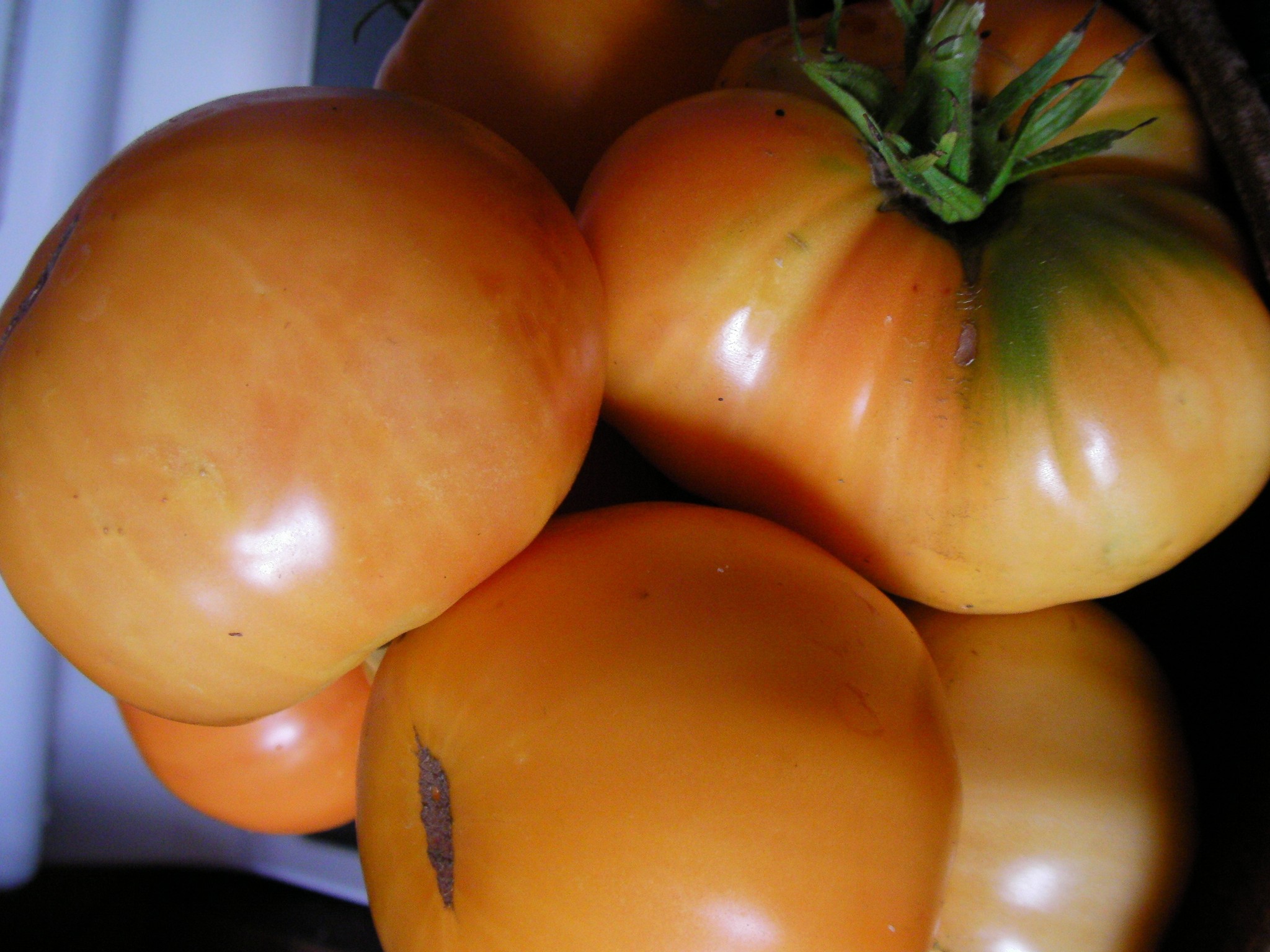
[0,0,1270,951]
